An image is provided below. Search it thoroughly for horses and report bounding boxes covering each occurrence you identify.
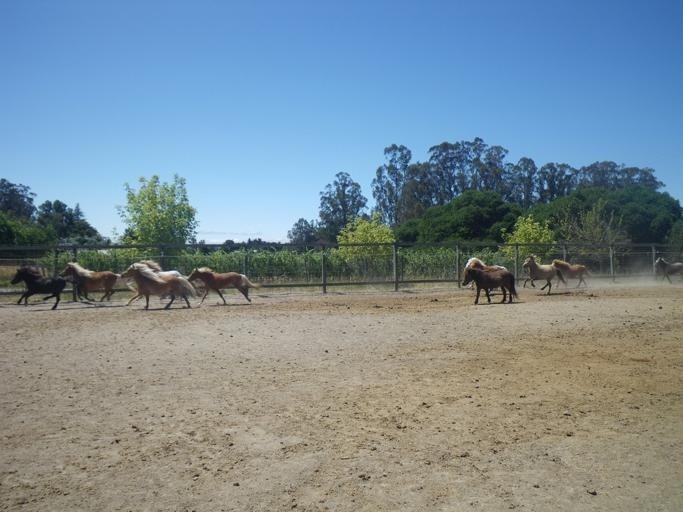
[462,256,519,305]
[10,265,77,310]
[552,259,593,289]
[58,261,121,302]
[653,257,683,284]
[121,259,261,310]
[523,254,566,294]
